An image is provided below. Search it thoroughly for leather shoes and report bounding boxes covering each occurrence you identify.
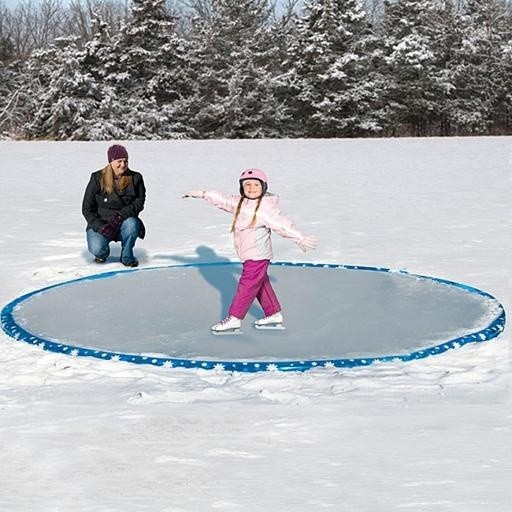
[95,247,110,264]
[120,257,139,268]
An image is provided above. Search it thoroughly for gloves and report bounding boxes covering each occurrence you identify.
[296,234,317,252]
[181,189,205,200]
[99,213,123,243]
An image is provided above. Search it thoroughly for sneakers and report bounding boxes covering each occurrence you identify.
[211,314,242,332]
[253,312,283,324]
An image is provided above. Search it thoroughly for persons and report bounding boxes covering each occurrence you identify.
[80,144,147,269]
[180,166,317,333]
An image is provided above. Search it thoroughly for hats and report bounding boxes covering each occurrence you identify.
[107,144,128,163]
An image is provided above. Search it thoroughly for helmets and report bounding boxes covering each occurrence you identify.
[239,168,268,192]
